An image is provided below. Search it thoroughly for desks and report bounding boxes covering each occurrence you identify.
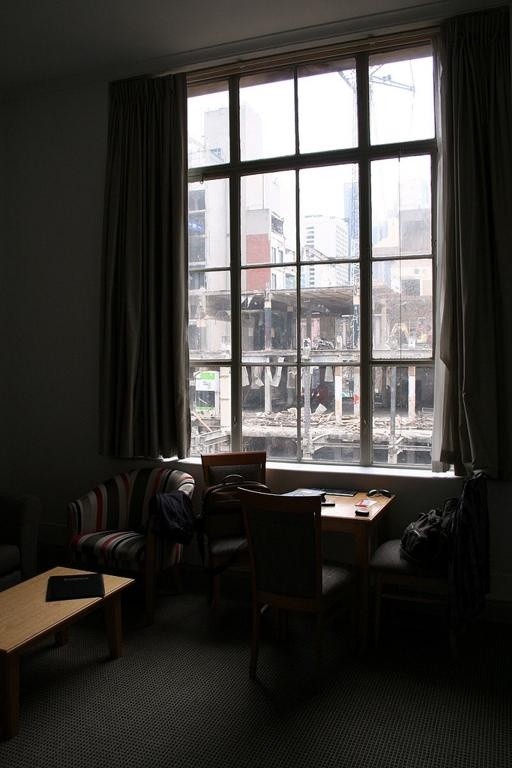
[0,565,137,740]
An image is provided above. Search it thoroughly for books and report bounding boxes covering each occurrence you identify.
[46,573,105,601]
[319,488,357,498]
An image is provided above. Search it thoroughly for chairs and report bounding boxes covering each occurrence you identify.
[0,492,42,587]
[198,452,491,689]
[67,466,198,626]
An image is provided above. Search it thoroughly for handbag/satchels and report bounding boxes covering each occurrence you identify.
[400,510,448,572]
[202,481,272,541]
